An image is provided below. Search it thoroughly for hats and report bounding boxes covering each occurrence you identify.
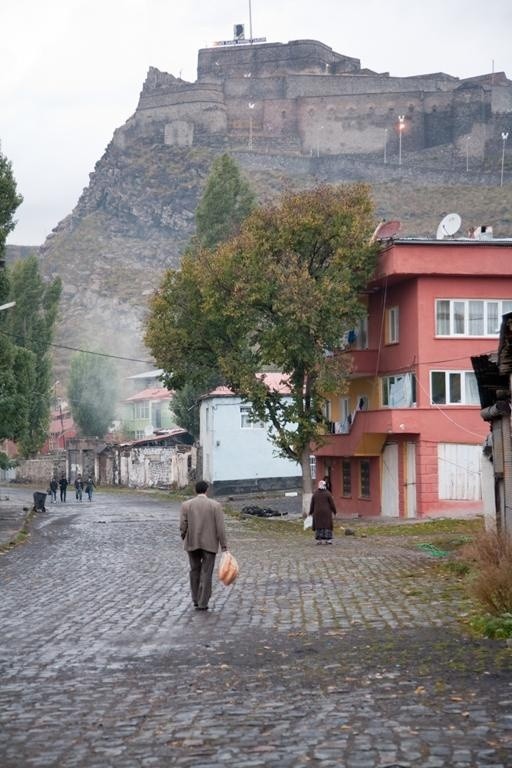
[318,480,327,490]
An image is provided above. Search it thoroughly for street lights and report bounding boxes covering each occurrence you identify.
[317,126,324,158]
[398,116,406,165]
[248,104,255,151]
[501,132,508,187]
[384,129,388,163]
[466,136,470,171]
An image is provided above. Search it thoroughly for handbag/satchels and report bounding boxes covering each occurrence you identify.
[304,515,312,531]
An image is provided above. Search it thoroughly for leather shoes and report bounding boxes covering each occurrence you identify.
[317,542,322,545]
[326,541,332,544]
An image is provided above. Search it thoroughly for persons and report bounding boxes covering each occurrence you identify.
[49,475,58,504]
[84,476,97,502]
[308,480,338,546]
[59,474,69,502]
[74,472,84,502]
[323,475,329,490]
[180,480,228,610]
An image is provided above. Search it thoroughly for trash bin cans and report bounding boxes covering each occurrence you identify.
[34,492,47,513]
